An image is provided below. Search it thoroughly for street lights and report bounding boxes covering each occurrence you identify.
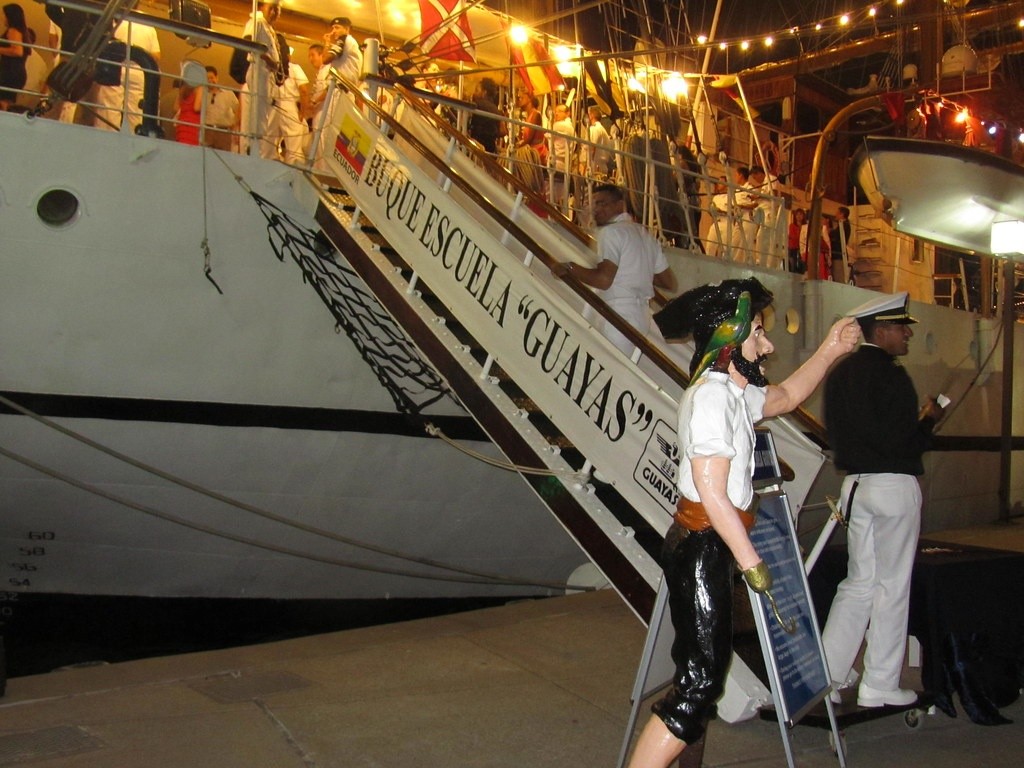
[990,218,1023,526]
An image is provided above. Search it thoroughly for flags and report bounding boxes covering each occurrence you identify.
[414,0,475,62]
[502,20,566,96]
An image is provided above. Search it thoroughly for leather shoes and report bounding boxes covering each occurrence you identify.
[830,685,842,705]
[855,680,918,708]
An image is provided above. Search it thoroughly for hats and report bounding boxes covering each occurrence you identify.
[480,78,498,98]
[330,17,351,27]
[848,292,920,328]
[263,0,282,6]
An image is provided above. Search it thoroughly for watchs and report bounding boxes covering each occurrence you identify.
[567,261,574,271]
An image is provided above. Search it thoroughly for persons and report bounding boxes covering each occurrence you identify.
[551,185,673,360]
[821,291,943,707]
[0,0,851,283]
[629,276,860,768]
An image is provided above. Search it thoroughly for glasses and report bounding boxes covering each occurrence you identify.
[210,93,216,104]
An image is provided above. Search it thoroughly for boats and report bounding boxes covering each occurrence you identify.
[1,0,1023,677]
[847,134,1024,258]
